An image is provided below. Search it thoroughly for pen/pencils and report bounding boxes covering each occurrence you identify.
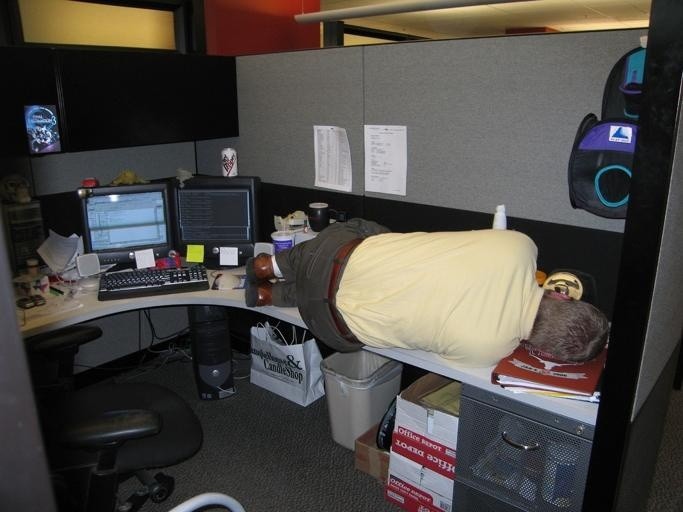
[50,286,65,295]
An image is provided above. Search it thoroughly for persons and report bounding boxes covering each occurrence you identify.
[245,217,610,367]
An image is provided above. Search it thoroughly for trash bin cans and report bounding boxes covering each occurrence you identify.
[320,351,402,451]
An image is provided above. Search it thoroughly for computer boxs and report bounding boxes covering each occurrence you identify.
[188,309,236,400]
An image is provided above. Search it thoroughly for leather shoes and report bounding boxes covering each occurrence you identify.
[245,281,273,308]
[245,252,274,282]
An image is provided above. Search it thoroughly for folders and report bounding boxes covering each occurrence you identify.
[491,344,609,397]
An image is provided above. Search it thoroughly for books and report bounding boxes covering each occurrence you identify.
[491,340,608,404]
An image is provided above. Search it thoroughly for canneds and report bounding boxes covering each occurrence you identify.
[270,231,295,255]
[28,260,39,274]
[308,203,330,232]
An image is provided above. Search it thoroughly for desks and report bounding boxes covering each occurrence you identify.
[17,252,609,430]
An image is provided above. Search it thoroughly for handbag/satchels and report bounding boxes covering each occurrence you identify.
[250,320,326,408]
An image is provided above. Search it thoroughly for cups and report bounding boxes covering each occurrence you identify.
[308,202,339,232]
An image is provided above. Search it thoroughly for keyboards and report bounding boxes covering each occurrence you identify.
[96,265,210,301]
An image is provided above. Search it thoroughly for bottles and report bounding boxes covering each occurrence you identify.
[491,202,508,231]
[221,147,238,177]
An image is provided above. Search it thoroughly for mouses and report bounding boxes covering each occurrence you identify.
[216,273,241,290]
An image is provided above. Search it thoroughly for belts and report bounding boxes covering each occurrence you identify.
[327,237,364,343]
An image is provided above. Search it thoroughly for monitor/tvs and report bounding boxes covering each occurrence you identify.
[174,177,258,257]
[80,183,173,258]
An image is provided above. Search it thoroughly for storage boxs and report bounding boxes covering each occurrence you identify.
[392,371,496,479]
[383,450,454,512]
[353,426,391,483]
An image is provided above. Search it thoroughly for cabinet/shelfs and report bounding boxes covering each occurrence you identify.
[452,384,593,512]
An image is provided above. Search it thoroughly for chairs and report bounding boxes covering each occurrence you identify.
[21,327,203,512]
